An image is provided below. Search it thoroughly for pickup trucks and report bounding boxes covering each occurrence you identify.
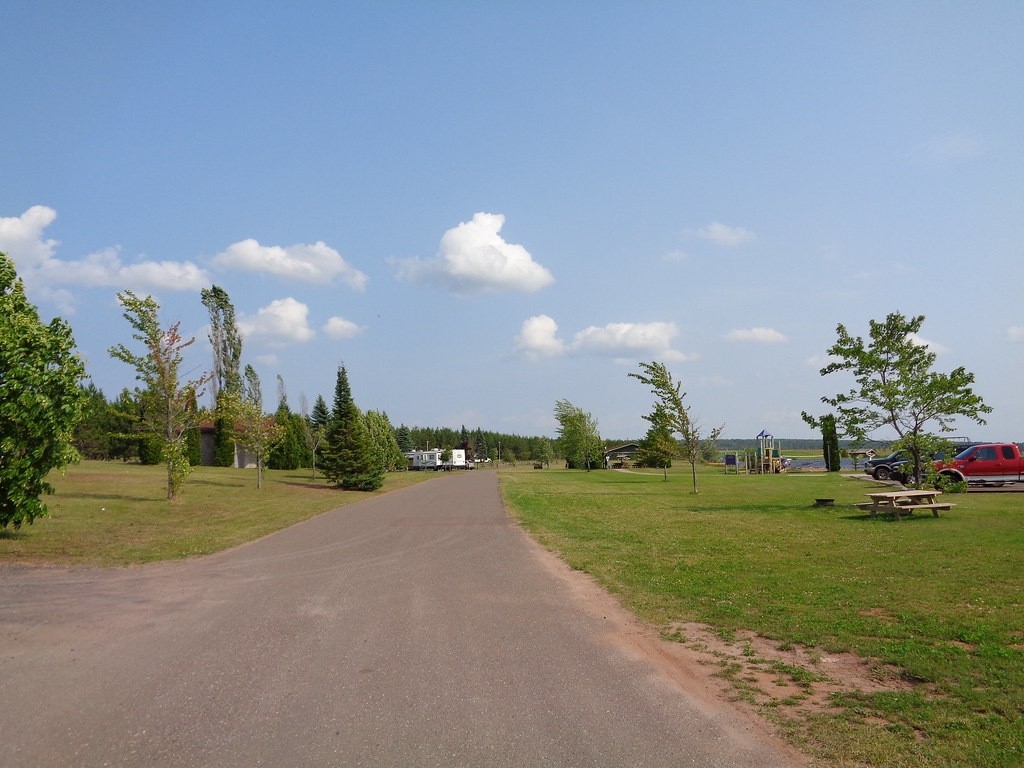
[924,442,1024,493]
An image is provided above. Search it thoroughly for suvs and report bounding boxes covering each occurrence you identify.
[889,441,1007,489]
[863,446,923,481]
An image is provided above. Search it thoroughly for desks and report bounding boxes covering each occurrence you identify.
[865,490,943,520]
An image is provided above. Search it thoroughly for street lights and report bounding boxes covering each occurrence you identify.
[498,442,502,464]
[426,441,429,452]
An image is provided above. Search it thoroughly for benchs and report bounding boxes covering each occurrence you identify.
[851,500,958,520]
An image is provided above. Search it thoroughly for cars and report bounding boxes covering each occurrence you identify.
[459,460,475,470]
